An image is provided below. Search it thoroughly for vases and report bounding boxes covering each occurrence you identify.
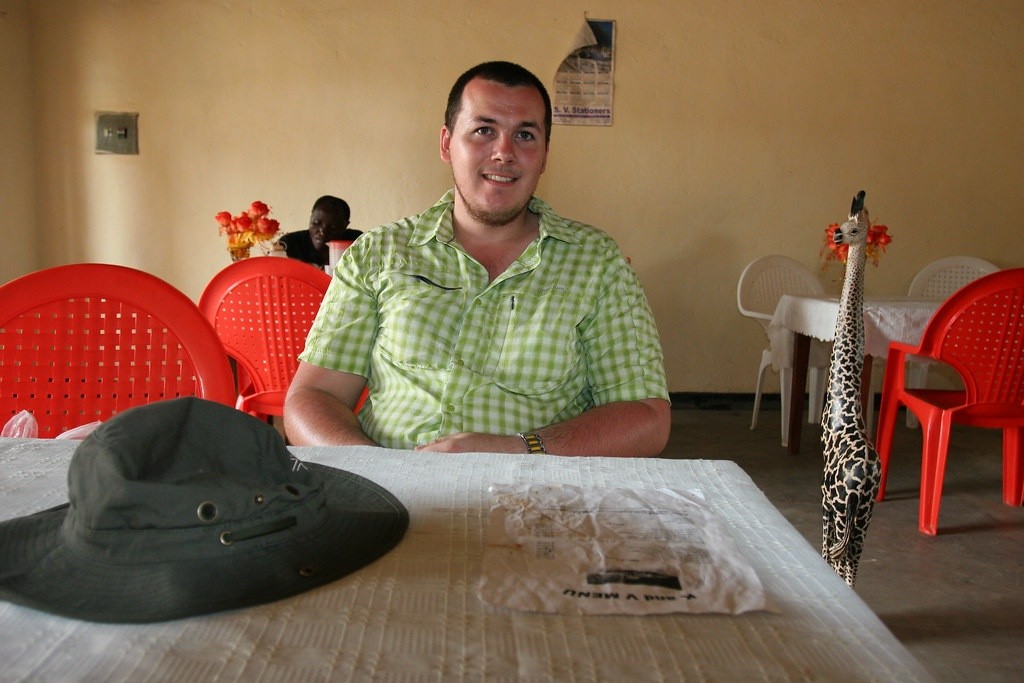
[230,248,250,261]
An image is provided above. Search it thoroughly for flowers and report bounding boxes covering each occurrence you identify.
[215,201,284,250]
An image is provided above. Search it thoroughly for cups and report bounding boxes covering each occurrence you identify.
[324,265,337,278]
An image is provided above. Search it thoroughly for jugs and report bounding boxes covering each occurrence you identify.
[325,240,354,266]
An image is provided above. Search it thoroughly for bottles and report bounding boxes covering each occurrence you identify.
[266,244,290,258]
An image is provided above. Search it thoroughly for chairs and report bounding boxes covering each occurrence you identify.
[906,255,1003,428]
[198,257,370,425]
[0,263,235,439]
[876,268,1024,536]
[740,254,873,446]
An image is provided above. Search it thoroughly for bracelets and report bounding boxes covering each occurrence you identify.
[517,432,545,454]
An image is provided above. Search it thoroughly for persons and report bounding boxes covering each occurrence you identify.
[268,195,363,268]
[284,61,671,456]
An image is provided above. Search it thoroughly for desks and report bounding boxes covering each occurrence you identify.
[0,437,939,683]
[768,294,945,454]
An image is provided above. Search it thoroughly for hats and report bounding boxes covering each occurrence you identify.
[1,397,410,625]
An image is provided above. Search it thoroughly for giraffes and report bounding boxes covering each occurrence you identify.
[820,190,881,590]
